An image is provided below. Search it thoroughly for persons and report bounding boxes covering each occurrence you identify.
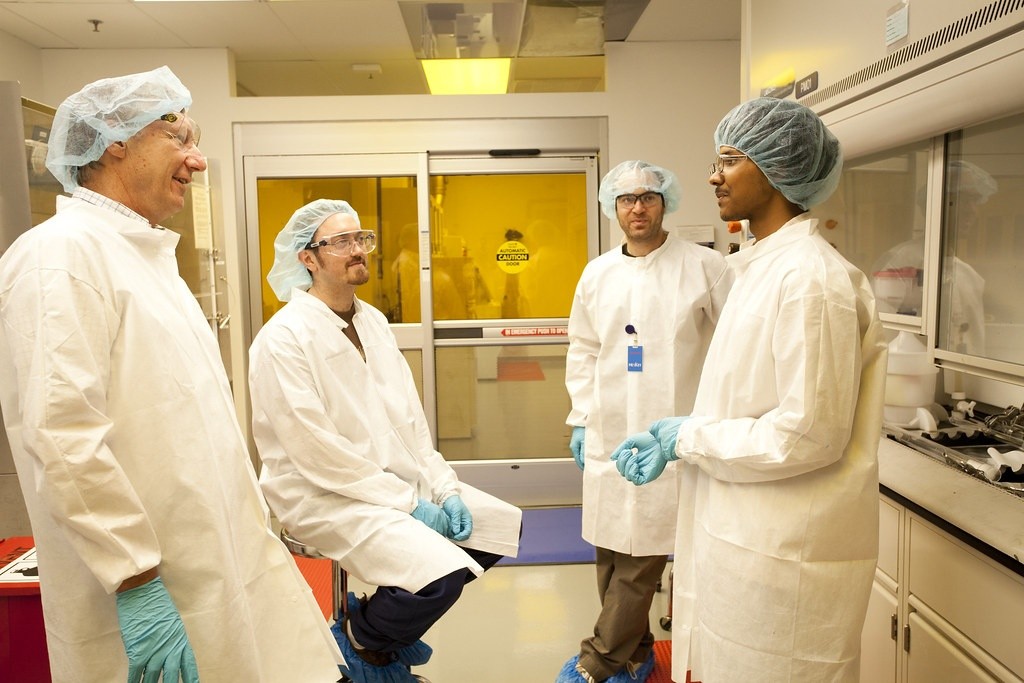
[556,162,736,683]
[0,65,349,683]
[870,160,999,357]
[610,97,888,683]
[387,218,577,467]
[248,199,524,683]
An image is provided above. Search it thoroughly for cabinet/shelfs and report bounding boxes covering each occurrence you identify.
[859,489,1024,683]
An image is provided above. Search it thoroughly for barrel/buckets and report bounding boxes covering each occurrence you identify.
[871,267,923,317]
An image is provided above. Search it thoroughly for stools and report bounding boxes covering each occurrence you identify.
[280,525,349,622]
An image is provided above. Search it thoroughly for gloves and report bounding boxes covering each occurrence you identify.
[569,426,585,471]
[648,415,692,462]
[443,494,473,542]
[116,576,200,683]
[609,431,667,486]
[411,496,453,539]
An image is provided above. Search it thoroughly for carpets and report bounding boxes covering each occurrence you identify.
[645,638,673,683]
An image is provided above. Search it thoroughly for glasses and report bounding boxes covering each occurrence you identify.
[305,229,378,258]
[707,154,754,177]
[156,110,202,152]
[616,190,666,209]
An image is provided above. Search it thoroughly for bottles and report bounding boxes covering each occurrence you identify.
[884,310,941,430]
